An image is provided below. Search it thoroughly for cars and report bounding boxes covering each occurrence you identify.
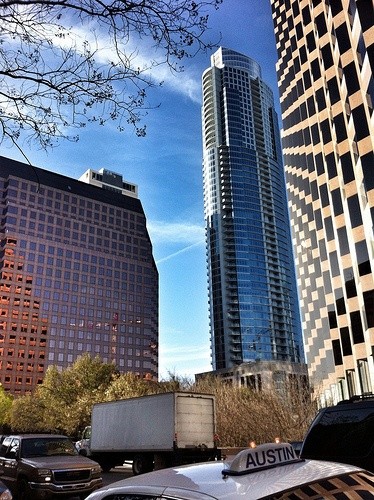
[0,433,104,500]
[85,442,374,500]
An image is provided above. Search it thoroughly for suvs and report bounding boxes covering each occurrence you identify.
[294,393,370,470]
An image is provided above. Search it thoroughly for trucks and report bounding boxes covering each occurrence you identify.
[76,390,221,475]
